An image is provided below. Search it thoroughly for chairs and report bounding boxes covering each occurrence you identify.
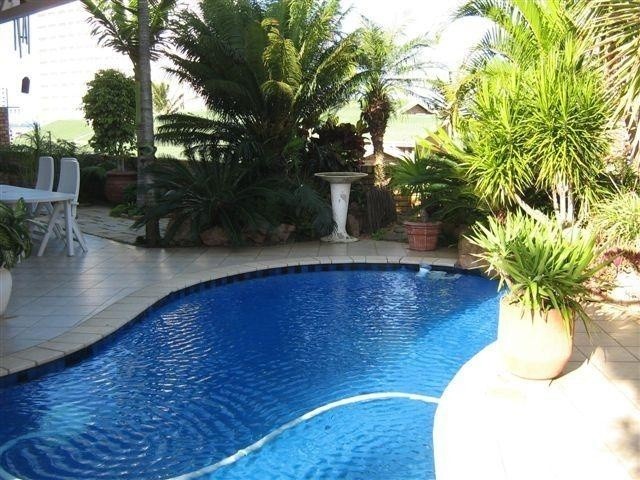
[32,156,59,256]
[34,158,88,256]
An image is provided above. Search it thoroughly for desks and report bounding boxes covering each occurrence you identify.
[1,181,76,258]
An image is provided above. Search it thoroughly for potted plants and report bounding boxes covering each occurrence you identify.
[0,194,49,322]
[79,67,145,205]
[459,204,628,383]
[379,140,451,252]
